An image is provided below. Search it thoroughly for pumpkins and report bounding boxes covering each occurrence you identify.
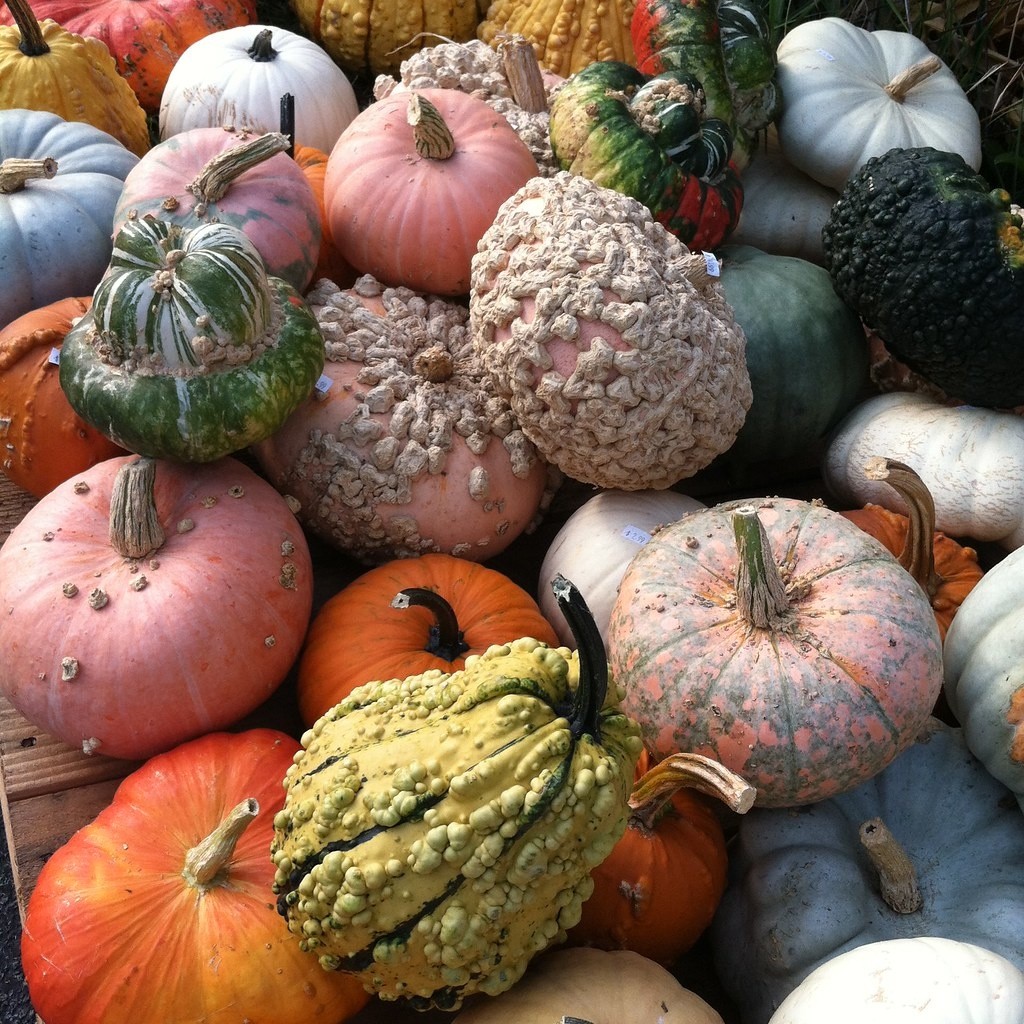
[0,0,1024,1024]
